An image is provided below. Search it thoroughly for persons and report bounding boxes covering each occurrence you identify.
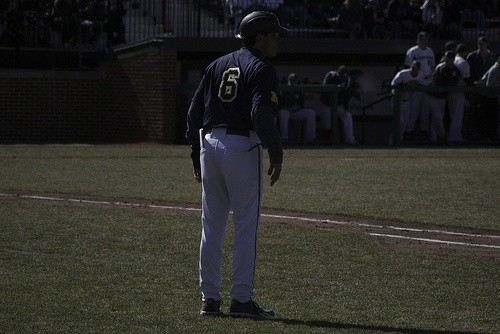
[194,0,500,41]
[390,31,500,145]
[0,0,127,50]
[187,11,292,319]
[319,65,358,145]
[277,72,320,148]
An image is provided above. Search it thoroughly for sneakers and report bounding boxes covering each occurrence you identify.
[230,297,275,319]
[201,298,223,315]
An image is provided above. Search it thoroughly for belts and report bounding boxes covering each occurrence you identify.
[202,127,250,138]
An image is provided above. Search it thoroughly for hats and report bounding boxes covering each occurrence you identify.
[239,11,291,37]
